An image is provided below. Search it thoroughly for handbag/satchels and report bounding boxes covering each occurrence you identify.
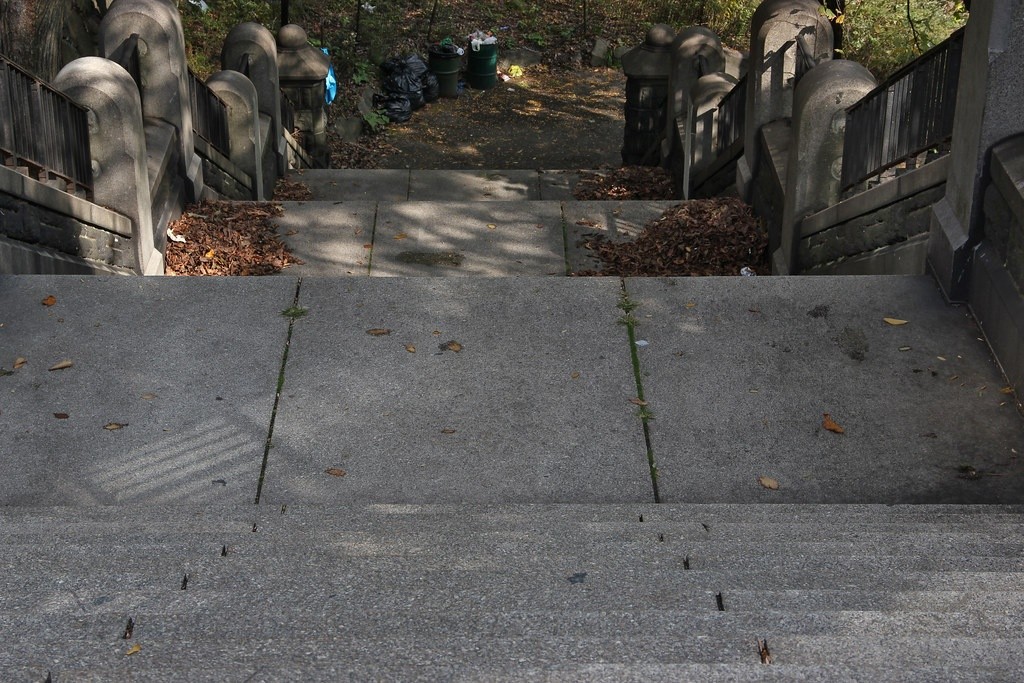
[321,47,337,106]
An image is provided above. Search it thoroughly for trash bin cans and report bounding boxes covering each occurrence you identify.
[429,44,461,98]
[468,40,499,90]
